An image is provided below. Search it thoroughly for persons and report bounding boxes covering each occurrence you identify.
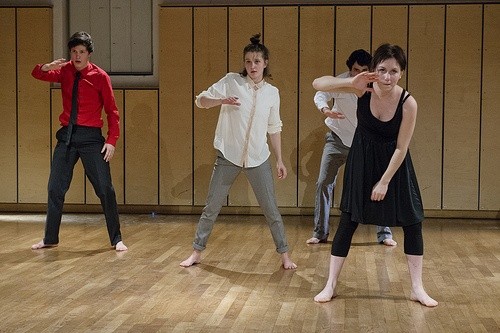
[306,49,398,245]
[312,41,439,308]
[30,31,128,250]
[180,33,298,269]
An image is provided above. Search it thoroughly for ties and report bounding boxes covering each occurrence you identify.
[65,71,82,147]
